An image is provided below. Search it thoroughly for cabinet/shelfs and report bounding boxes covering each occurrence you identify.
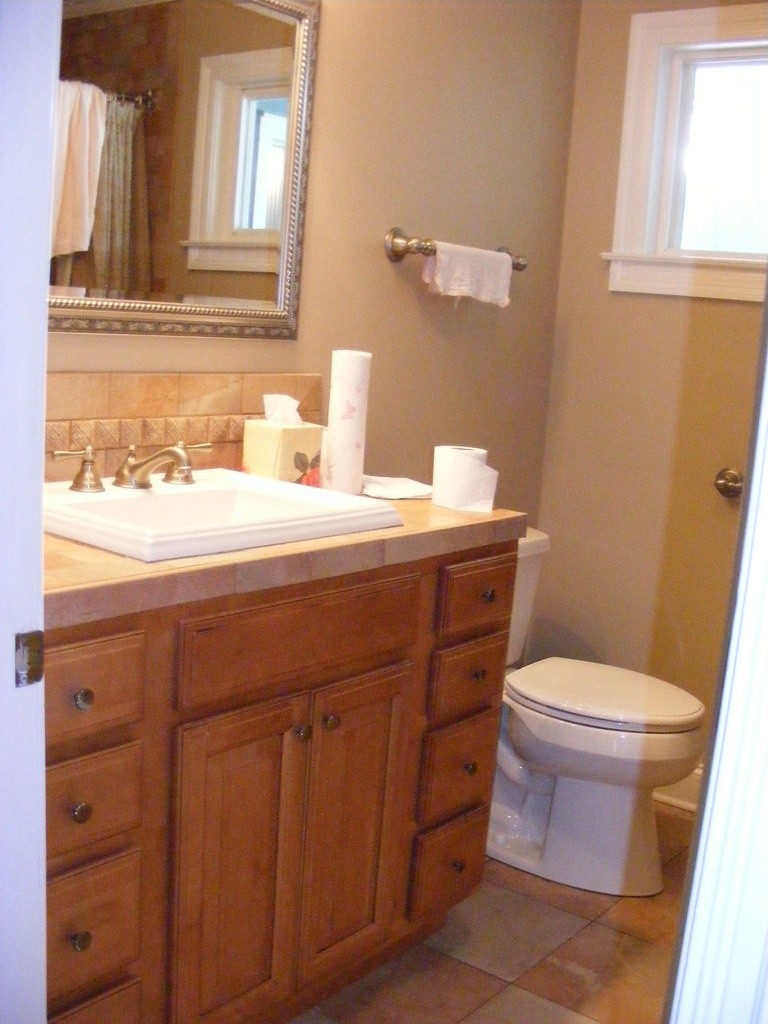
[43,466,528,1020]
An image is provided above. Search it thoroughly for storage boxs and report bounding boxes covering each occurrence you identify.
[242,419,328,489]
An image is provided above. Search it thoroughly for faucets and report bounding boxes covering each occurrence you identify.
[111,440,195,490]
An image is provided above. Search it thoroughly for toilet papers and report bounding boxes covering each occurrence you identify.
[431,441,501,515]
[322,349,372,495]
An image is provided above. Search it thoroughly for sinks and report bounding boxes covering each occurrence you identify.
[40,467,405,567]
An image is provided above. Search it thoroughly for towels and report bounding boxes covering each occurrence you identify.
[358,472,436,502]
[420,239,514,311]
[50,80,107,262]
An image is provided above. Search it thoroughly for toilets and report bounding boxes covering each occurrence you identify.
[485,526,710,898]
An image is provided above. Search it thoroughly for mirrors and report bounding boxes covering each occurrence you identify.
[48,0,300,308]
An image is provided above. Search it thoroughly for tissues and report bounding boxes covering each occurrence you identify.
[239,392,335,492]
